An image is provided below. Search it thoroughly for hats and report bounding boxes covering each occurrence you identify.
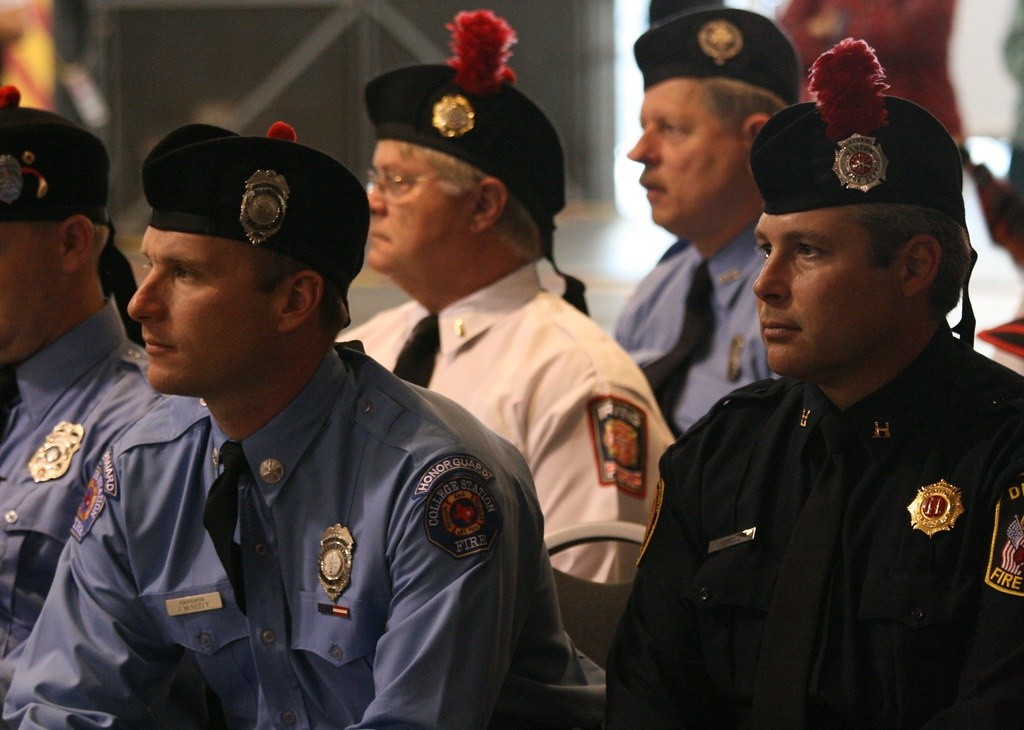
[142,123,371,285]
[0,85,109,223]
[750,36,964,227]
[634,8,802,104]
[364,8,566,237]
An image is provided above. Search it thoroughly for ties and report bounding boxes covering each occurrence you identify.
[643,260,716,421]
[202,442,252,615]
[392,315,437,388]
[748,407,872,730]
[0,363,22,449]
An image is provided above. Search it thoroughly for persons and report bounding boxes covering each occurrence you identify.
[0,85,162,730]
[2,119,607,730]
[605,38,1024,730]
[609,11,800,439]
[334,11,676,663]
